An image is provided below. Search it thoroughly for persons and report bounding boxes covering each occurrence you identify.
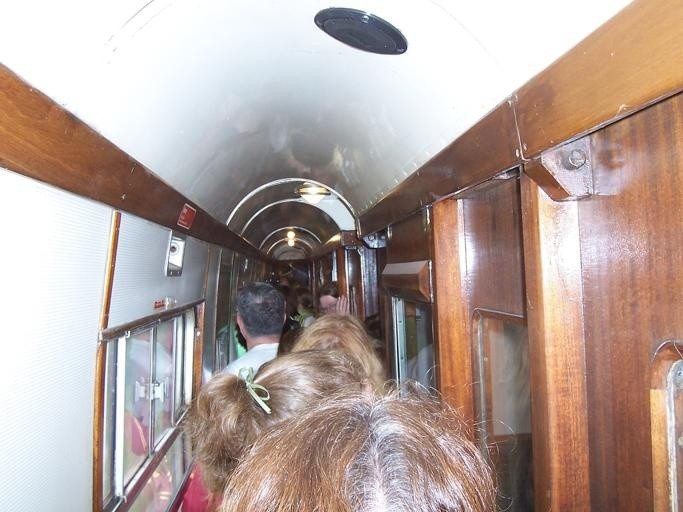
[203,375,502,512]
[178,278,386,512]
